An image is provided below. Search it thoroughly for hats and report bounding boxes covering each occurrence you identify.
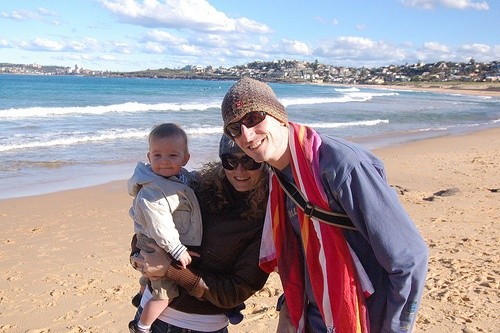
[219,133,244,158]
[222,75,289,141]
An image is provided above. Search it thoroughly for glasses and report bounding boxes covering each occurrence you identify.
[222,155,261,170]
[224,110,269,137]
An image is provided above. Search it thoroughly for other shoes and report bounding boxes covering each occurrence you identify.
[128,320,152,332]
[132,292,142,307]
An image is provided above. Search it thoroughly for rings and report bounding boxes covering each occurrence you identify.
[142,267,144,271]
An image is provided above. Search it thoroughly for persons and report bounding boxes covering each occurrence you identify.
[129,132,270,333]
[126,121,204,332]
[222,76,429,332]
